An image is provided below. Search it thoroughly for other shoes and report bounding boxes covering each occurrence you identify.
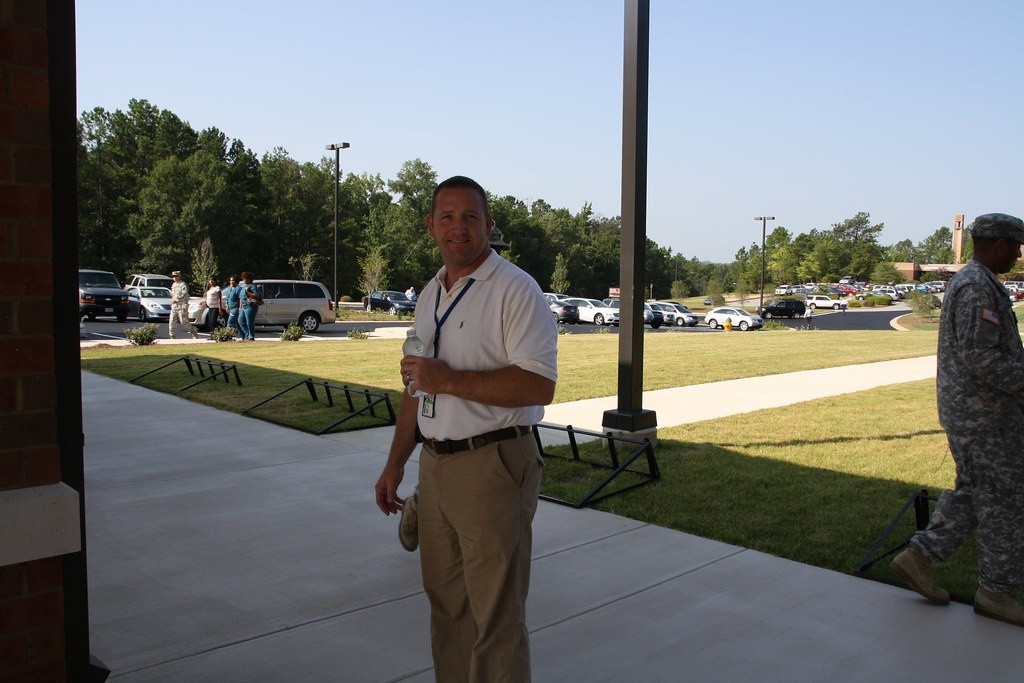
[170,337,175,339]
[192,330,198,339]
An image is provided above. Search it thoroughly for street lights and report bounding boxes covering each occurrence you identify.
[754,217,775,308]
[325,143,350,315]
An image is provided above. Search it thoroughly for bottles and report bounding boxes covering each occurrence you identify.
[402,330,428,397]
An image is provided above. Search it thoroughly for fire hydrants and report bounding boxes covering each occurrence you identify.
[724,317,732,332]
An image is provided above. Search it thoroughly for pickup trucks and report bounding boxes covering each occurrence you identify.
[775,285,792,296]
[839,276,855,284]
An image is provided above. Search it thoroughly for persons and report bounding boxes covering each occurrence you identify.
[238,271,259,341]
[169,271,197,340]
[891,214,1024,627]
[375,177,558,683]
[840,297,848,315]
[225,275,244,341]
[399,227,511,553]
[804,306,813,330]
[206,275,223,334]
[405,286,416,300]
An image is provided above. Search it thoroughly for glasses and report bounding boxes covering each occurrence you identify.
[173,275,179,277]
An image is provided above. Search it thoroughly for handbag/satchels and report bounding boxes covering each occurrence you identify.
[245,283,261,307]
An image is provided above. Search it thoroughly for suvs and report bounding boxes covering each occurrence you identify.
[124,274,175,289]
[79,269,131,322]
[805,295,840,310]
[755,299,805,319]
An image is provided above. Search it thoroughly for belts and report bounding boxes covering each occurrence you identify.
[421,425,533,456]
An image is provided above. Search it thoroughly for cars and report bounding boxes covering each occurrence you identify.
[364,291,420,316]
[543,293,699,329]
[705,306,763,331]
[1002,281,1024,302]
[703,295,725,305]
[125,286,173,323]
[792,281,948,301]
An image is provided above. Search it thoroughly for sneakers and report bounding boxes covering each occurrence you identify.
[399,495,418,552]
[889,547,950,607]
[974,586,1024,627]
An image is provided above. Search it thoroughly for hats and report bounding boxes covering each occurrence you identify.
[972,213,1024,245]
[172,271,181,276]
[489,227,511,251]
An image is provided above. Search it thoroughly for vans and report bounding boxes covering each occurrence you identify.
[188,279,335,333]
